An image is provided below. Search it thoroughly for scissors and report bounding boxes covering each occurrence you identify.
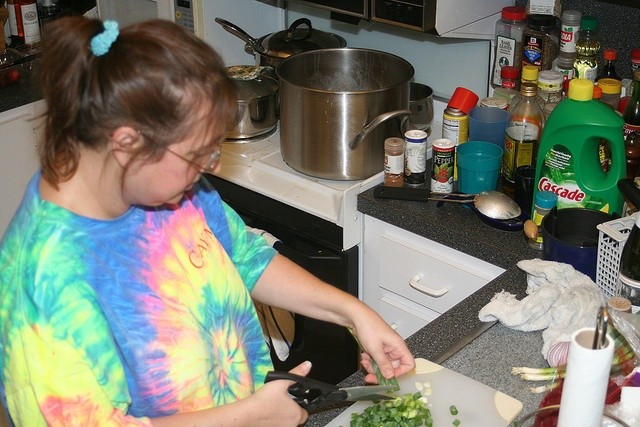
[264,371,397,415]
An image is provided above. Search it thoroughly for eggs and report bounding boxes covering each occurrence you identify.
[524,218,538,238]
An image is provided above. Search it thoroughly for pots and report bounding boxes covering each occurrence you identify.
[224,66,277,139]
[215,17,347,83]
[276,47,414,180]
[410,82,434,130]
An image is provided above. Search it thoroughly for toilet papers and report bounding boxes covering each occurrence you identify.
[555,326,617,427]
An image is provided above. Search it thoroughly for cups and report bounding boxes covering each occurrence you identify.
[514,165,536,201]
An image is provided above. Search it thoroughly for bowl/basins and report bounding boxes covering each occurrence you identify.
[542,208,616,283]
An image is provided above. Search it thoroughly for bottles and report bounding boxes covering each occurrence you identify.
[594,86,609,173]
[573,16,601,83]
[559,78,573,102]
[628,47,640,79]
[560,10,581,57]
[520,13,560,71]
[623,69,640,167]
[598,78,619,107]
[511,66,545,129]
[490,6,526,89]
[619,95,632,113]
[533,191,557,232]
[492,65,518,110]
[616,213,640,306]
[597,49,624,81]
[502,82,546,198]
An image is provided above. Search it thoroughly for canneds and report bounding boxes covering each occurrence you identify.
[404,128,427,187]
[432,139,455,194]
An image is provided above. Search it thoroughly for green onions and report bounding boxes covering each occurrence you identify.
[512,330,636,394]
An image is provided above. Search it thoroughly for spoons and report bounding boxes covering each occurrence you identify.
[374,186,523,220]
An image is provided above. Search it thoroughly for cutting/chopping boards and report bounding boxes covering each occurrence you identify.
[325,357,523,426]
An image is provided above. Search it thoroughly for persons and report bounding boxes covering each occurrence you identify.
[0,13,417,427]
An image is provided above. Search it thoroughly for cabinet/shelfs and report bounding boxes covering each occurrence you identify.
[360,213,505,350]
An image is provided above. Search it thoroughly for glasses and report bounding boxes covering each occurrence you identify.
[134,128,223,172]
